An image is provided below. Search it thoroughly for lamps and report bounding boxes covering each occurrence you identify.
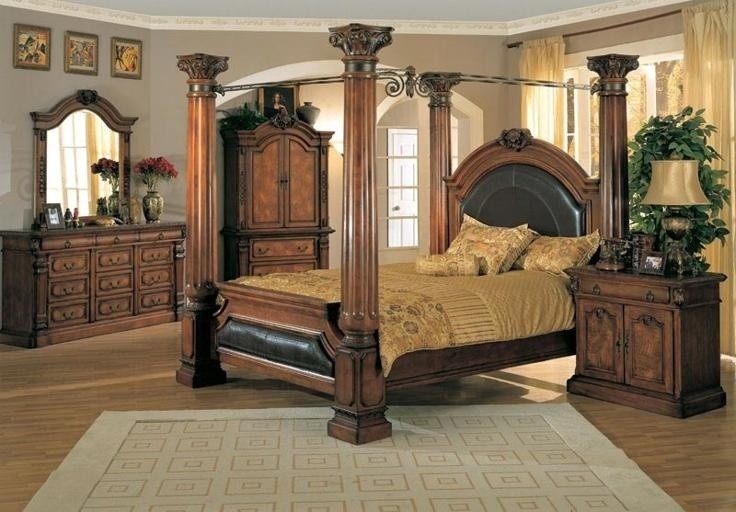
[639,159,714,279]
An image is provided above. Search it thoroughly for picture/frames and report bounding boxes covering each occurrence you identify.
[637,249,669,276]
[40,200,67,229]
[110,36,143,79]
[63,30,100,76]
[12,24,53,71]
[258,82,300,121]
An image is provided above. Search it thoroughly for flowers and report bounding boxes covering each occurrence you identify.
[131,153,178,192]
[90,156,119,191]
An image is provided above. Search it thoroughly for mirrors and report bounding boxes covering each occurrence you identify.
[29,89,139,229]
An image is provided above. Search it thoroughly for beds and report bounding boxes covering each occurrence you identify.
[174,20,643,448]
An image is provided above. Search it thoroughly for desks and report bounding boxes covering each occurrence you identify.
[0,220,189,351]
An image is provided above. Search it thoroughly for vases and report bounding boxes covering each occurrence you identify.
[106,190,119,215]
[295,99,321,128]
[143,190,164,224]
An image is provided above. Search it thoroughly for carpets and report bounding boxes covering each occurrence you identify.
[17,399,687,510]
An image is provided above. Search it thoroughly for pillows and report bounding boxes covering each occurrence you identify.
[411,251,482,277]
[510,228,603,280]
[455,237,514,276]
[443,212,542,273]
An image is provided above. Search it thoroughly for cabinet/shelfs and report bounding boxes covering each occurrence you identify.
[217,106,336,281]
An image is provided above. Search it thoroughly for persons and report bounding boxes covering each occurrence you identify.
[50,209,59,224]
[645,258,660,269]
[270,92,289,117]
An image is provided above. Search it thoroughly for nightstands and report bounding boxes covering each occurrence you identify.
[561,263,728,420]
